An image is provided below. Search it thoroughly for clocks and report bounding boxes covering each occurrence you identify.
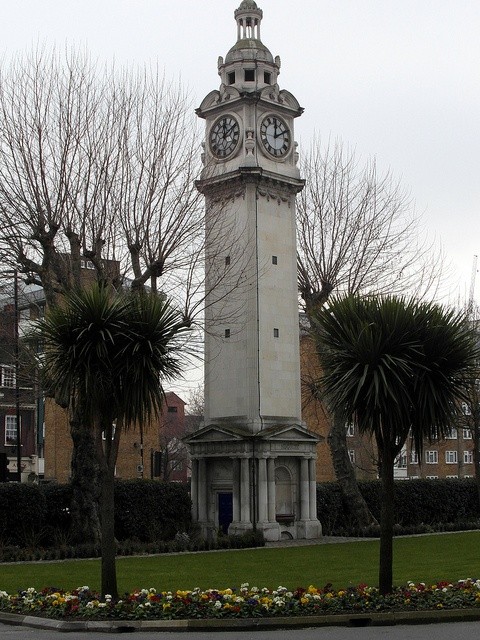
[207,111,244,163]
[256,112,294,162]
